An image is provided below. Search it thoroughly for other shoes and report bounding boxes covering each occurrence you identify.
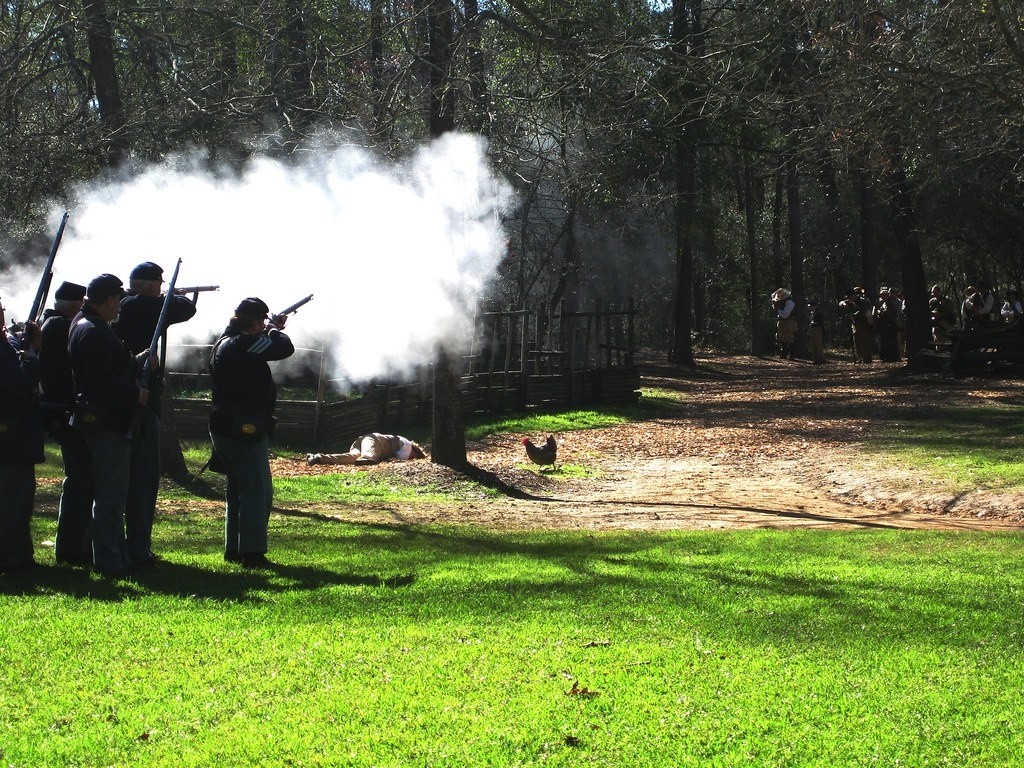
[306,453,318,466]
[356,457,376,466]
[224,551,245,564]
[127,546,177,573]
[97,565,145,584]
[243,555,279,569]
[58,554,93,569]
[780,346,908,367]
[0,556,51,576]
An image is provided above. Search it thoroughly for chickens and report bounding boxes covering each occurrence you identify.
[521,435,557,471]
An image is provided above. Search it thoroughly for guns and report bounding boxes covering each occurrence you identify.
[124,258,182,441]
[16,211,71,365]
[175,285,222,294]
[267,292,315,329]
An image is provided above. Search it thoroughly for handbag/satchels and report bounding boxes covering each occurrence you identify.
[68,401,106,432]
[227,416,265,440]
[0,416,20,443]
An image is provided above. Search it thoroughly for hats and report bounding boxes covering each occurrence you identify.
[968,279,992,290]
[87,274,123,296]
[56,282,86,301]
[238,298,269,318]
[130,262,163,281]
[879,287,889,295]
[897,291,905,298]
[843,288,860,299]
[806,297,820,306]
[1009,291,1019,300]
[771,288,792,302]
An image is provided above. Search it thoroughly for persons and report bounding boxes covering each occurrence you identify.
[306,432,427,467]
[109,262,196,572]
[961,281,1024,329]
[872,286,908,362]
[1,298,46,593]
[771,288,800,360]
[40,280,95,564]
[808,300,826,365]
[929,285,952,351]
[209,296,295,569]
[838,287,878,365]
[66,273,159,577]
